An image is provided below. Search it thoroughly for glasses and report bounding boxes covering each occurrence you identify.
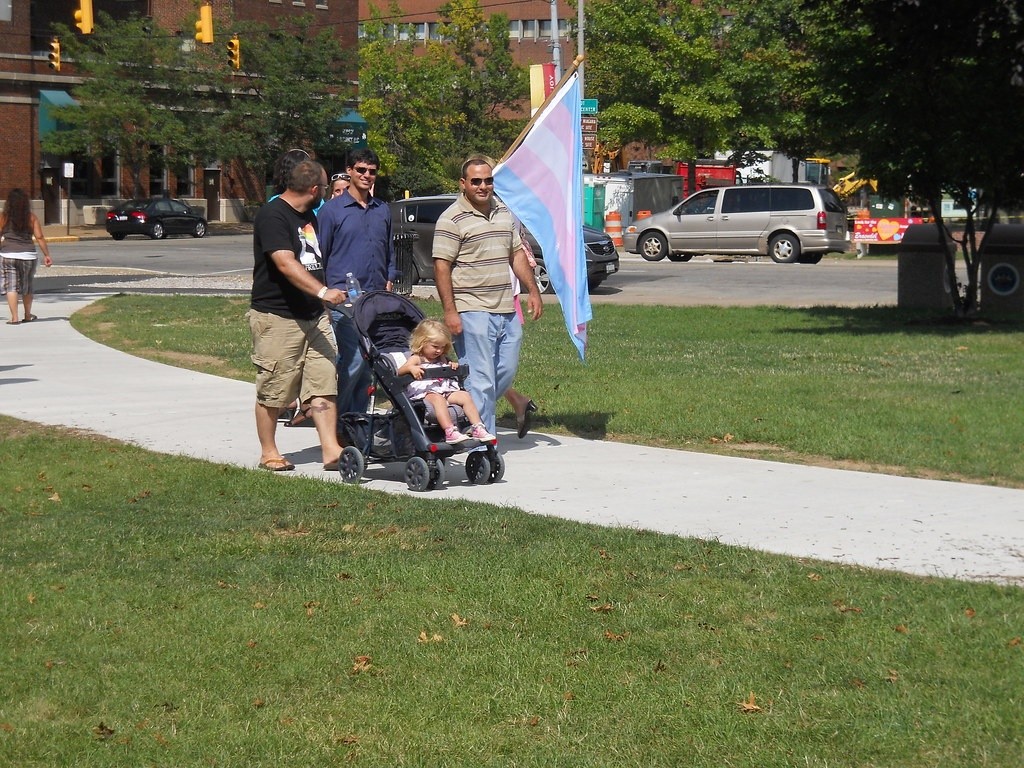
[466,176,494,186]
[331,173,351,181]
[313,183,329,190]
[352,166,378,175]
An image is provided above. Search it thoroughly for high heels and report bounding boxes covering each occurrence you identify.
[518,398,539,438]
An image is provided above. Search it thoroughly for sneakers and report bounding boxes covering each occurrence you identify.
[444,426,469,445]
[471,423,497,442]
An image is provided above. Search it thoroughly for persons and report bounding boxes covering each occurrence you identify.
[0,187,53,324]
[250,159,346,471]
[397,319,496,444]
[267,148,395,448]
[431,154,544,451]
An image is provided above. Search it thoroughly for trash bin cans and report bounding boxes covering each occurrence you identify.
[870,194,900,217]
[979,224,1024,320]
[898,225,957,310]
[391,232,414,297]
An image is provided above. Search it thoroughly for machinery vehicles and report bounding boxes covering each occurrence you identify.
[803,158,881,203]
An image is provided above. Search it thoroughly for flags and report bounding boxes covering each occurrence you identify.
[491,72,593,361]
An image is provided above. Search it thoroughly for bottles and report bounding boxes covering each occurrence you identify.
[345,272,362,303]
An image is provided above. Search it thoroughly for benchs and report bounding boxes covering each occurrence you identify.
[852,218,924,258]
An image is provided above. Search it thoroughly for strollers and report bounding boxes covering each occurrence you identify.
[333,289,514,491]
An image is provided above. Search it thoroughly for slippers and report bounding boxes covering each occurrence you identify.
[323,458,339,471]
[22,315,37,323]
[258,456,295,471]
[6,320,20,325]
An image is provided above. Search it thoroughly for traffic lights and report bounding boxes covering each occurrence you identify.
[74,0,94,36]
[226,40,240,70]
[195,6,215,44]
[47,41,61,72]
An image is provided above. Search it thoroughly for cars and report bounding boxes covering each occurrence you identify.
[623,185,864,261]
[106,197,208,241]
[380,194,616,296]
